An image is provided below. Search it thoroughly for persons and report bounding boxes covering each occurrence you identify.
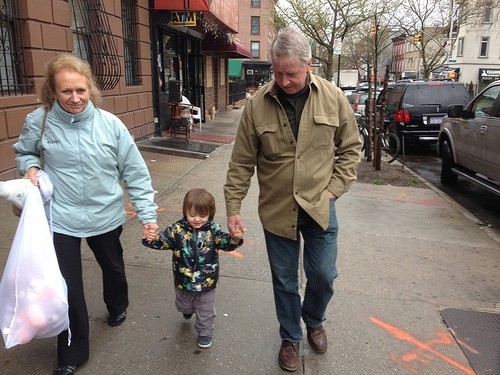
[142,188,243,348]
[12,54,159,375]
[223,29,361,371]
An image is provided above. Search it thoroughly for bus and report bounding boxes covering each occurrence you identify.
[335,69,359,90]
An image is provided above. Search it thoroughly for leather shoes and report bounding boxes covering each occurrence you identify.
[53,363,78,375]
[107,301,128,327]
[278,339,300,372]
[306,324,327,354]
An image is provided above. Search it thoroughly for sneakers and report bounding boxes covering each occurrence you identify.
[197,335,213,348]
[183,313,193,319]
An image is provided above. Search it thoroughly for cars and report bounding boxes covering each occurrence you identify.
[376,78,472,153]
[439,79,500,203]
[348,92,381,119]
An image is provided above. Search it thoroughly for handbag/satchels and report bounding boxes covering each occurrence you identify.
[0,170,73,349]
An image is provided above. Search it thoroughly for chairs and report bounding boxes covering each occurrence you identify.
[170,95,201,141]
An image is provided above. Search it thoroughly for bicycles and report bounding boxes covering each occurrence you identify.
[353,112,399,163]
